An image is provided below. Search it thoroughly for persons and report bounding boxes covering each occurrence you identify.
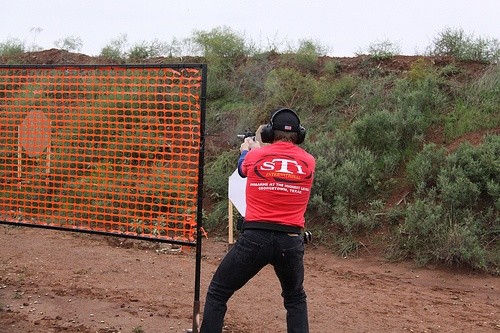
[198,107,315,333]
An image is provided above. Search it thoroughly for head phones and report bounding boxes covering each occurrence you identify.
[260,108,306,144]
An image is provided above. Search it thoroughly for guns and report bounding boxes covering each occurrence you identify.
[236,131,256,141]
